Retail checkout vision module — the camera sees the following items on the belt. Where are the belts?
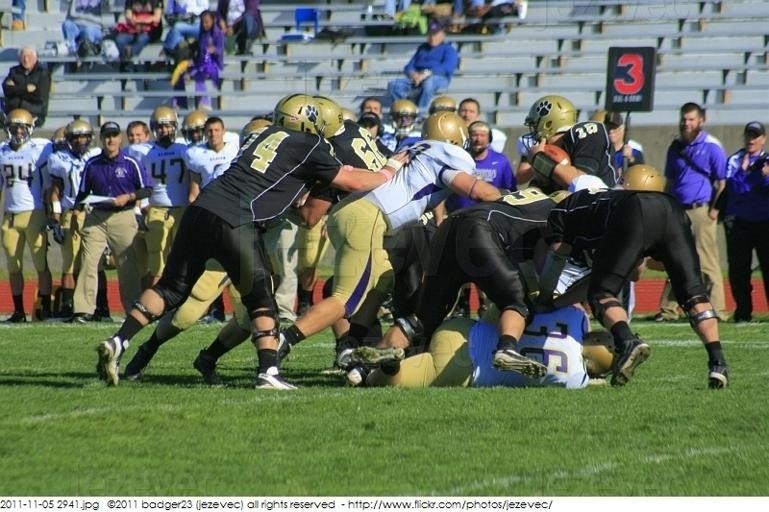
[681,202,709,209]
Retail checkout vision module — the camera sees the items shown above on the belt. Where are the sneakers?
[124,370,142,381]
[253,330,300,392]
[332,343,406,386]
[192,348,225,387]
[0,310,115,324]
[707,361,730,389]
[652,312,753,323]
[490,347,550,379]
[95,334,131,388]
[609,337,652,388]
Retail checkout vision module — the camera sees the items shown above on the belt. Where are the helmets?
[581,329,618,379]
[51,119,96,142]
[466,119,494,151]
[390,98,420,118]
[522,94,580,142]
[591,110,625,131]
[148,105,179,130]
[421,94,471,151]
[271,92,343,141]
[237,118,274,149]
[613,163,666,196]
[180,110,208,132]
[3,108,34,126]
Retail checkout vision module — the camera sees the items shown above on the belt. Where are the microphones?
[480,144,491,152]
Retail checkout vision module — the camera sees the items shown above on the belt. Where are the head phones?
[357,112,384,137]
[467,120,493,142]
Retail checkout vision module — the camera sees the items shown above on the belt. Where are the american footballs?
[544,143,572,168]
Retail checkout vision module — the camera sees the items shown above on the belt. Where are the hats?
[356,111,381,125]
[100,122,120,135]
[428,21,441,33]
[745,120,766,137]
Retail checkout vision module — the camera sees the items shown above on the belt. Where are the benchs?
[0,1,769,132]
